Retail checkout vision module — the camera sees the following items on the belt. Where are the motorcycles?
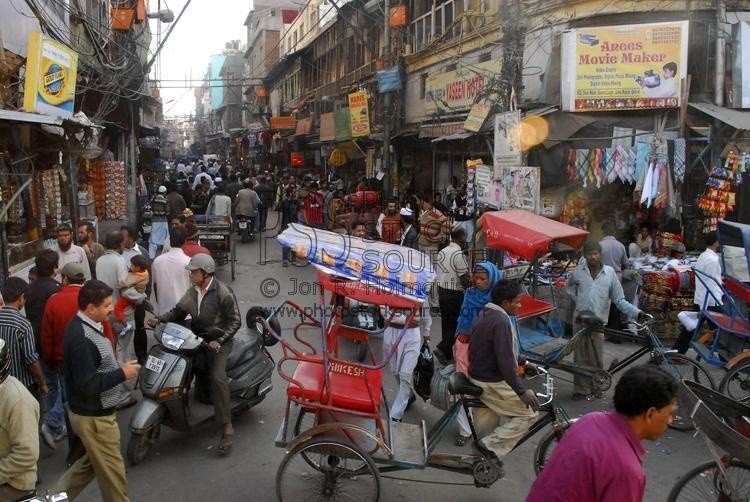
[17,479,70,502]
[234,201,263,244]
[123,298,280,468]
[140,207,152,243]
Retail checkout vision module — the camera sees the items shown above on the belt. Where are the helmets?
[184,253,215,274]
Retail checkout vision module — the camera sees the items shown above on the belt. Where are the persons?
[523,363,678,501]
[640,63,678,97]
[45,278,143,500]
[0,337,41,500]
[1,159,727,467]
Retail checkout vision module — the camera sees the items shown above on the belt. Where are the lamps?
[149,8,173,26]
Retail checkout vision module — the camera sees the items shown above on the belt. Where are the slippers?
[219,434,234,455]
[472,439,506,472]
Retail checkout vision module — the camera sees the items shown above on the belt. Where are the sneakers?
[434,349,455,366]
[39,423,68,450]
[120,322,132,336]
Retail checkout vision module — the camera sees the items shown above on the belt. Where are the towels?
[630,158,679,211]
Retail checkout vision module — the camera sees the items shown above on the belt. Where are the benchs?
[188,214,231,240]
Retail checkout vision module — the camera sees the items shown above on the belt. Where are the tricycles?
[194,214,239,282]
[264,207,750,502]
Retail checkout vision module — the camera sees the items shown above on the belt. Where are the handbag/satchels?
[431,364,456,411]
[413,341,434,402]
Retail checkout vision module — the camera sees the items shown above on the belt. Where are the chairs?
[503,272,556,327]
[689,268,750,340]
[260,298,383,415]
[714,221,750,305]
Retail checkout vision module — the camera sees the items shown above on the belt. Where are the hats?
[185,223,200,240]
[56,262,85,279]
[215,177,222,182]
[399,208,412,215]
[159,185,166,193]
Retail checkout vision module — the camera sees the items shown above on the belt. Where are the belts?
[389,322,419,329]
